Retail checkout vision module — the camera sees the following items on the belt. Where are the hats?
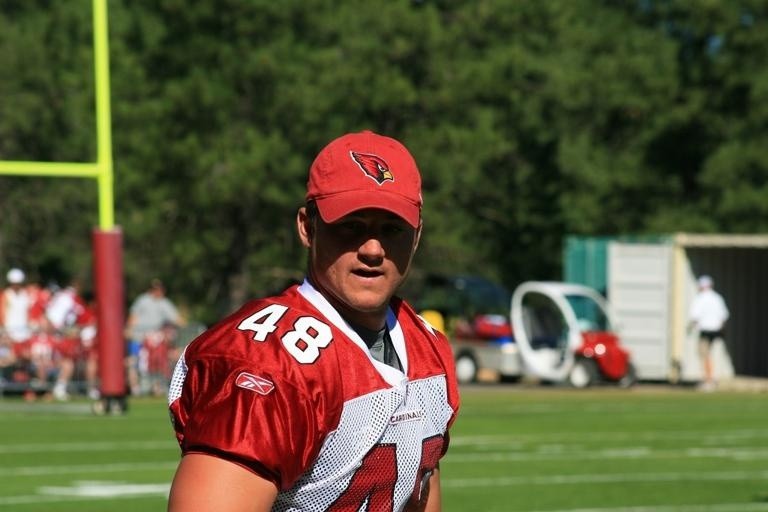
[697,274,714,289]
[6,267,27,285]
[304,128,424,229]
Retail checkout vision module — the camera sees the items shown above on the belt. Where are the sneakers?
[695,380,723,393]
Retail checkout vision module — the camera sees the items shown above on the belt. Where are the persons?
[686,276,729,389]
[0,265,182,406]
[170,130,461,512]
